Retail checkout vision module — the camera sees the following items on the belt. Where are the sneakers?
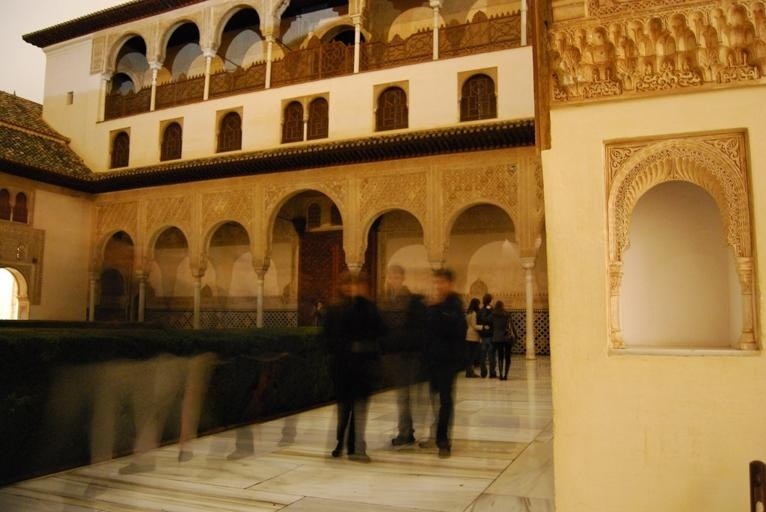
[391,432,416,446]
[418,438,451,459]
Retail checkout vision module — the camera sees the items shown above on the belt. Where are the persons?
[319,264,467,465]
[465,293,516,380]
[84,329,298,499]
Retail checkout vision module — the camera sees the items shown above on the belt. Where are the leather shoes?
[465,372,507,380]
[331,439,367,459]
